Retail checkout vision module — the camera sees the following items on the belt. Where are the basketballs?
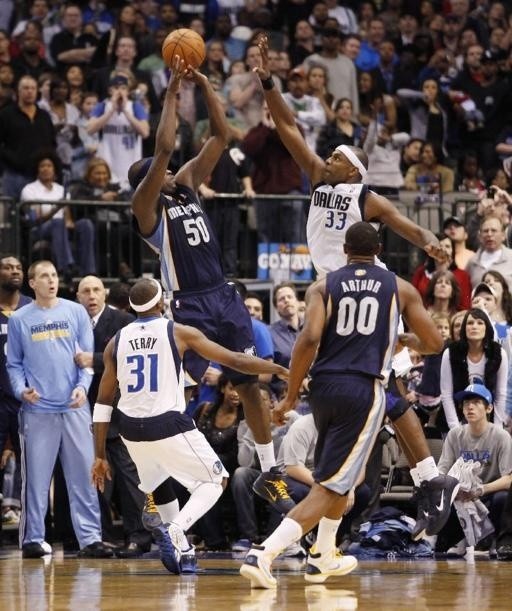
[161,28,206,73]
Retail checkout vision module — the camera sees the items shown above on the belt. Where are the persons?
[1,2,512,590]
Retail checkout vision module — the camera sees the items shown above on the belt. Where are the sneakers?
[231,538,255,552]
[419,470,462,536]
[76,541,114,560]
[141,490,164,533]
[445,538,469,558]
[252,462,297,514]
[151,520,186,575]
[180,543,206,573]
[305,530,317,545]
[305,585,358,611]
[239,541,278,591]
[114,538,151,559]
[1,510,21,530]
[21,540,46,558]
[494,543,512,561]
[411,485,431,544]
[284,541,307,558]
[304,542,359,583]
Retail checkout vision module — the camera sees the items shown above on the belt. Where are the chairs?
[379,437,400,499]
[384,439,445,501]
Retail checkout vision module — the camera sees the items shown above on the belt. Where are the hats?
[107,66,137,89]
[471,282,498,302]
[455,383,493,406]
[479,48,498,64]
[287,67,306,82]
[442,215,468,233]
